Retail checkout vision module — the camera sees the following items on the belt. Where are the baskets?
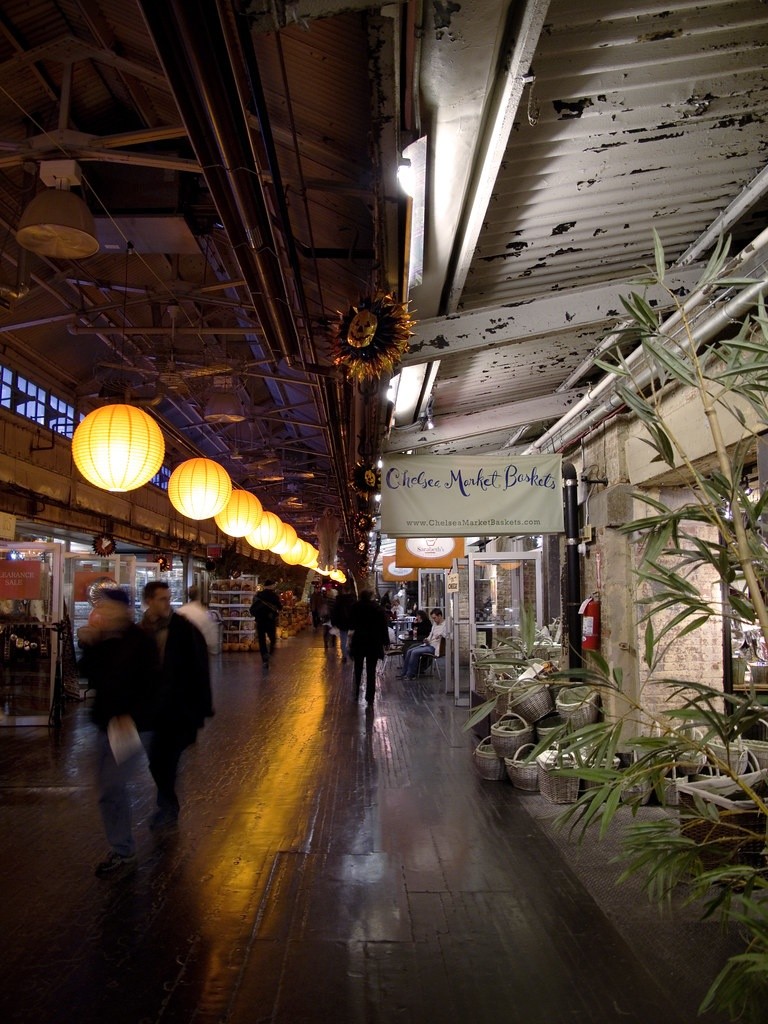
[677,768,768,894]
[472,636,722,805]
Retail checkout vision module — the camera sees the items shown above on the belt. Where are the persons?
[381,583,407,628]
[0,600,75,718]
[310,585,359,664]
[77,580,219,875]
[249,580,283,670]
[396,608,446,681]
[350,590,389,704]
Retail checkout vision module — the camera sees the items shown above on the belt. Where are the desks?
[399,634,425,641]
[392,621,417,631]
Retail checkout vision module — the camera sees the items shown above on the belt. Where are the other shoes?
[365,703,374,712]
[395,674,416,680]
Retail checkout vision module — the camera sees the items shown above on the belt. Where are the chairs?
[377,636,447,682]
[404,616,417,631]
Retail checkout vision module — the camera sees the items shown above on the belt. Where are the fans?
[98,305,233,395]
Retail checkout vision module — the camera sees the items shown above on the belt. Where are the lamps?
[419,406,434,432]
[281,539,347,583]
[268,523,297,555]
[245,511,284,550]
[167,423,232,520]
[213,490,263,537]
[257,449,284,481]
[70,239,164,492]
[16,160,100,259]
[203,373,246,422]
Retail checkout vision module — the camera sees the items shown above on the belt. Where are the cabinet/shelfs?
[208,578,255,644]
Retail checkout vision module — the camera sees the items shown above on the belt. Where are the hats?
[263,578,274,586]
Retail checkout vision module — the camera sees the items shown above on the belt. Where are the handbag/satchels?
[732,652,745,684]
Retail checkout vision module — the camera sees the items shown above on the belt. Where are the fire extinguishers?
[582,591,601,650]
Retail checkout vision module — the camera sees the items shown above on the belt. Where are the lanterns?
[245,512,347,583]
[214,490,263,538]
[71,404,166,493]
[168,458,232,521]
